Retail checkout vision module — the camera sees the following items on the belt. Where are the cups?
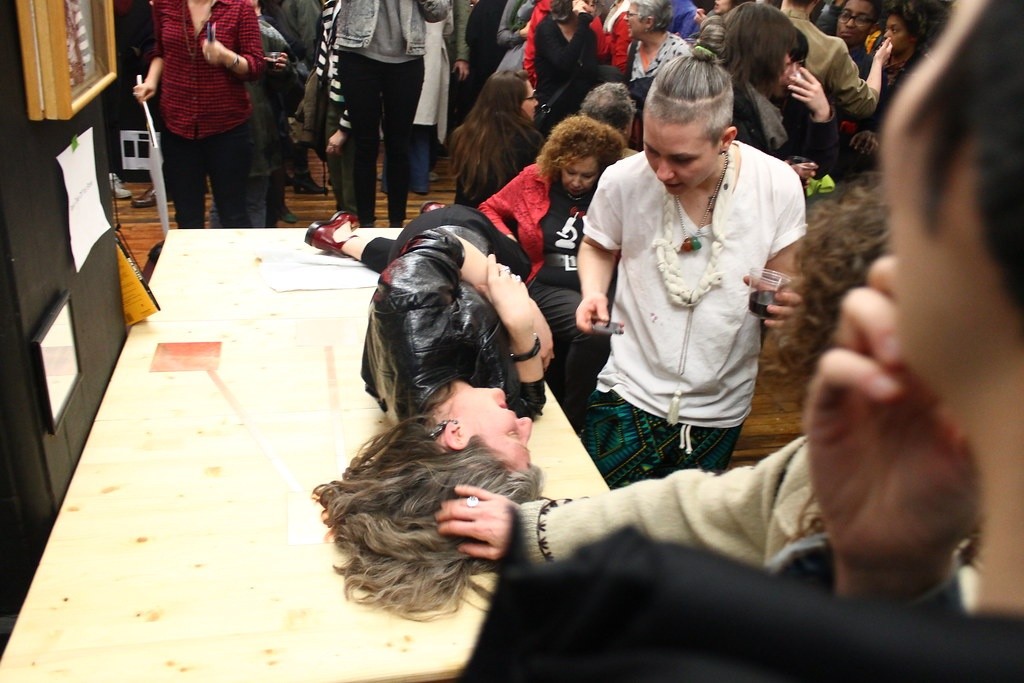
[747,267,791,321]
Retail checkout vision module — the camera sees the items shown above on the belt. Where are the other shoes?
[277,203,297,224]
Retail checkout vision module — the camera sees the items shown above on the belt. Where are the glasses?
[838,10,874,28]
[626,11,639,18]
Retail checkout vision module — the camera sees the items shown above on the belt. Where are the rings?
[467,495,479,508]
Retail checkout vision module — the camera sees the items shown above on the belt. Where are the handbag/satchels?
[624,76,655,114]
[531,88,551,134]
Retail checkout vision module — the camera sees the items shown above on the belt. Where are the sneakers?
[109,173,131,200]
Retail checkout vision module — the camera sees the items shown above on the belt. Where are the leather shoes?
[130,186,170,208]
[306,209,361,258]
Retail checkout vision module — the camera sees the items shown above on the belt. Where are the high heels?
[292,172,328,195]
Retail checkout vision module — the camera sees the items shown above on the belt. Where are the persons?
[304,202,556,621]
[432,176,986,590]
[108,0,1024,492]
[441,0,1022,683]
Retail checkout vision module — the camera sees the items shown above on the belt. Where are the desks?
[2,224,630,681]
